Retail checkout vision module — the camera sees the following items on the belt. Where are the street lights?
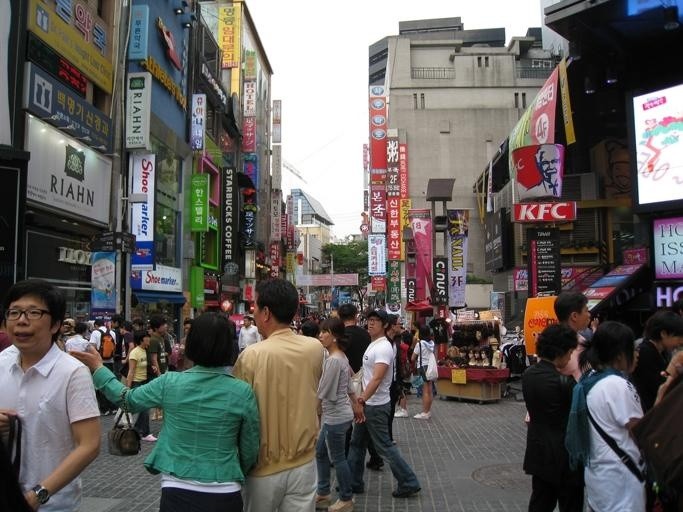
[422,176,455,258]
[402,224,417,279]
[115,187,148,315]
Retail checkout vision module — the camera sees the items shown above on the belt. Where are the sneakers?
[392,489,421,497]
[141,434,157,441]
[394,409,409,417]
[413,412,432,420]
[367,462,383,467]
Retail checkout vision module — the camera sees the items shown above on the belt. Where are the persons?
[2,280,101,511]
[335,309,421,499]
[69,312,260,510]
[459,338,502,368]
[126,330,159,442]
[55,314,194,415]
[411,325,436,419]
[232,278,323,511]
[316,319,365,511]
[522,145,562,198]
[384,313,400,446]
[237,316,262,353]
[290,312,332,337]
[521,291,683,512]
[335,304,384,470]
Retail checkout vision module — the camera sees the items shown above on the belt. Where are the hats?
[367,310,388,322]
[489,338,499,346]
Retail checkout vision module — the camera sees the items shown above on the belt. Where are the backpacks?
[95,328,115,360]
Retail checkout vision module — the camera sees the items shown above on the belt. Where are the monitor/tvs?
[624,75,683,213]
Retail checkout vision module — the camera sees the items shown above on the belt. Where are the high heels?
[315,493,331,509]
[328,499,354,512]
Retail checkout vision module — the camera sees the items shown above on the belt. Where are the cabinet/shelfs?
[434,367,510,404]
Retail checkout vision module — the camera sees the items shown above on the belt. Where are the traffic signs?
[85,231,113,252]
[120,232,136,252]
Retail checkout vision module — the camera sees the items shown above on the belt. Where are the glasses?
[3,308,52,320]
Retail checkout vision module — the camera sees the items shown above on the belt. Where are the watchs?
[32,484,50,504]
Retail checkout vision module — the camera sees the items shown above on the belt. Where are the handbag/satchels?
[419,352,439,382]
[108,388,139,456]
[0,414,34,511]
